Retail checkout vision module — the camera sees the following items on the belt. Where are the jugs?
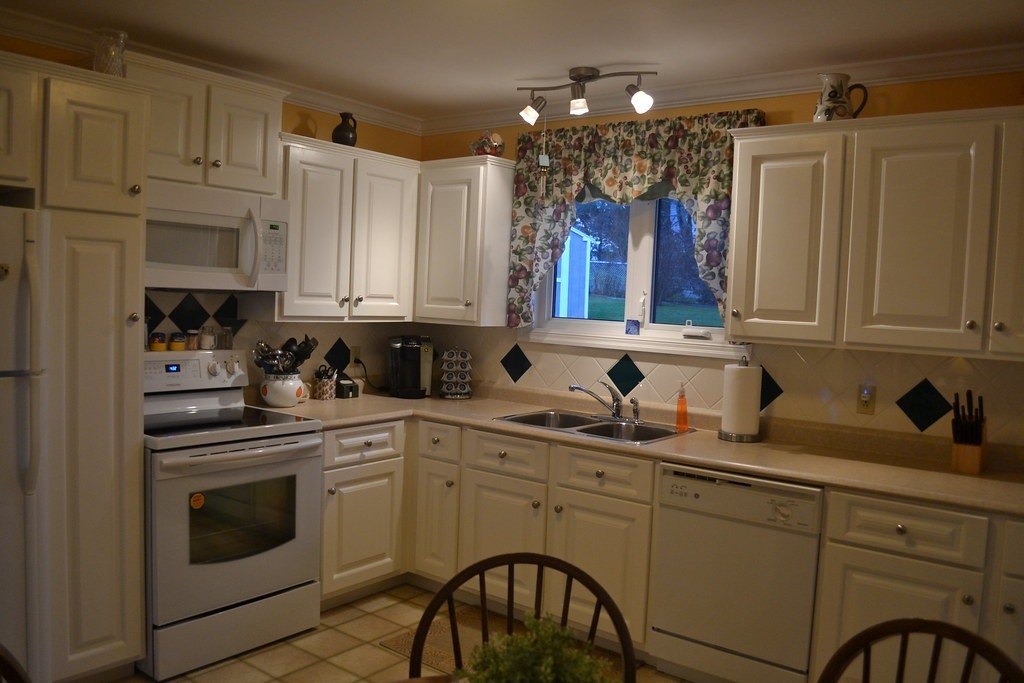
[332,113,357,147]
[94,29,127,79]
[813,73,868,123]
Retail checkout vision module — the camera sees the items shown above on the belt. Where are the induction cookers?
[142,352,323,450]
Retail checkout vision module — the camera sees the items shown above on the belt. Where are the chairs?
[815,619,1023,683]
[412,554,635,683]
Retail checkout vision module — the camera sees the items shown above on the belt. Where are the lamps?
[516,67,658,126]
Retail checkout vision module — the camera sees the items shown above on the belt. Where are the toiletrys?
[676,381,688,434]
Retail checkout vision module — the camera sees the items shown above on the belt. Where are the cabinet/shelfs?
[817,486,1023,683]
[413,155,517,328]
[409,418,462,587]
[121,49,288,198]
[850,104,1024,364]
[463,426,658,654]
[17,211,147,683]
[321,420,409,602]
[237,128,421,324]
[724,120,851,351]
[37,53,149,219]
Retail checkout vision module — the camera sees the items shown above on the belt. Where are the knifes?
[952,389,984,444]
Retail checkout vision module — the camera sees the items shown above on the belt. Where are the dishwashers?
[646,460,824,683]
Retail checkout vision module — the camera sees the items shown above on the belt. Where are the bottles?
[219,327,233,349]
[677,382,688,433]
[187,330,199,350]
[169,333,185,351]
[201,326,217,349]
[261,373,303,407]
[151,332,167,351]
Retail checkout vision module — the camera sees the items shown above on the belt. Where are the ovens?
[145,432,323,681]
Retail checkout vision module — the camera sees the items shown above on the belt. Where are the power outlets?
[856,384,876,415]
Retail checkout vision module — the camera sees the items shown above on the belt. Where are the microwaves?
[143,206,288,292]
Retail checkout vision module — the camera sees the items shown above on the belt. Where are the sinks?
[576,424,678,446]
[491,408,606,428]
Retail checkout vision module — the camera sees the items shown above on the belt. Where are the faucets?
[569,384,615,414]
[629,396,639,421]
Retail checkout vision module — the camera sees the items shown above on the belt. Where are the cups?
[313,378,336,399]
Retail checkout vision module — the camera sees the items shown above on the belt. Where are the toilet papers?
[720,364,763,435]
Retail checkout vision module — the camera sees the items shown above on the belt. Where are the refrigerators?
[0,205,82,681]
